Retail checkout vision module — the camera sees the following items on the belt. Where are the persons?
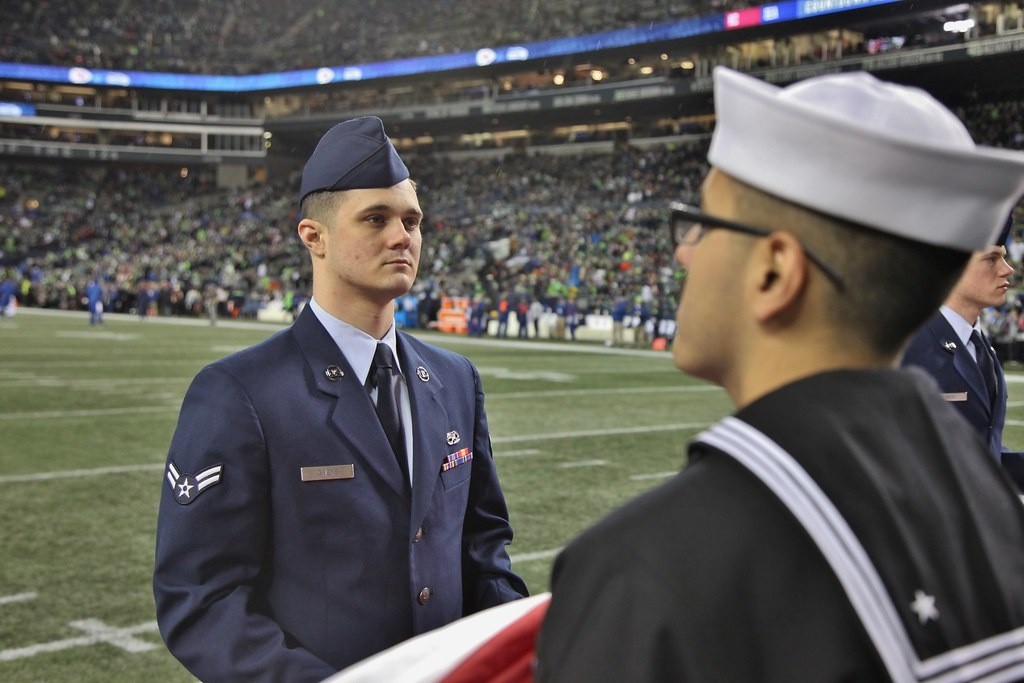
[536,62,1024,683]
[901,206,1014,470]
[153,120,532,683]
[0,0,1024,371]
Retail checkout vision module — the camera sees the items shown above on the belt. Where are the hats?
[996,206,1013,246]
[702,64,1024,254]
[281,116,411,210]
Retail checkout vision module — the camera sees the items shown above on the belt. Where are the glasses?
[667,199,845,295]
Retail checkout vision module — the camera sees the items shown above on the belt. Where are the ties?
[373,343,410,482]
[970,329,998,414]
